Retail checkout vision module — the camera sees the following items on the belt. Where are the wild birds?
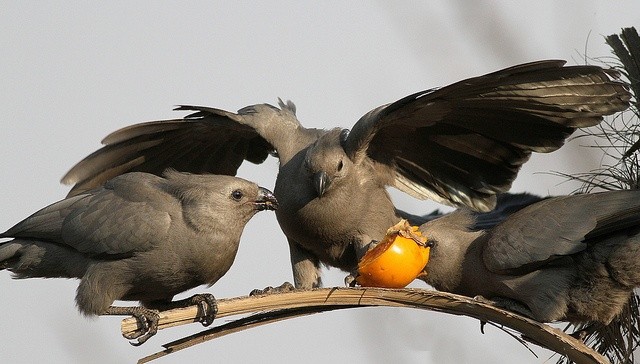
[413,186,639,337]
[57,59,634,296]
[0,168,279,349]
